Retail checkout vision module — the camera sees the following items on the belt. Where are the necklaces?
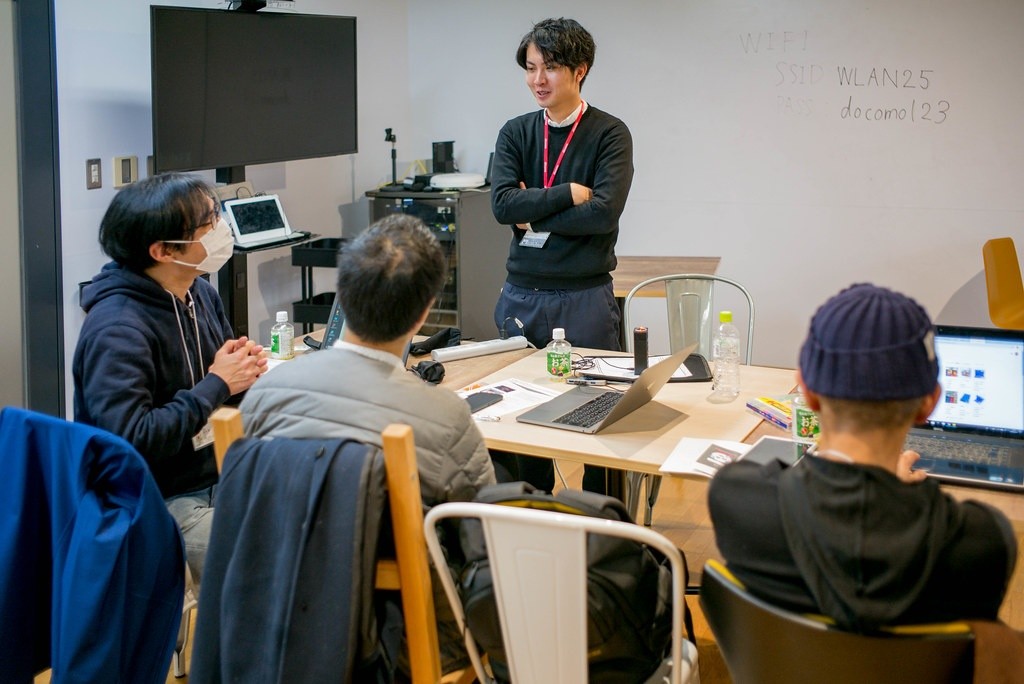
[814,449,852,463]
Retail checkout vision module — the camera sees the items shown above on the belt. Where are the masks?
[158,218,235,272]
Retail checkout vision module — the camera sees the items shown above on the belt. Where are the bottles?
[546,327,572,379]
[270,311,296,360]
[791,383,822,441]
[710,310,741,401]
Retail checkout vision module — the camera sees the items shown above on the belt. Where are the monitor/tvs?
[149,5,358,175]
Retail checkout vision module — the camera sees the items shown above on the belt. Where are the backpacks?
[458,479,688,684]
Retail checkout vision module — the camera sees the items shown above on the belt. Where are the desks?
[610,255,723,351]
[242,317,1024,633]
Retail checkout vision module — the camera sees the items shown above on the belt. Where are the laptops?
[319,289,412,368]
[901,325,1024,493]
[516,342,700,434]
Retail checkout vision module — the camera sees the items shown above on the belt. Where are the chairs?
[0,407,201,684]
[697,556,1023,684]
[983,237,1024,329]
[208,407,489,683]
[624,271,752,529]
[424,501,699,684]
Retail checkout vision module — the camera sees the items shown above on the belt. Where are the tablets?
[224,195,292,245]
[739,435,817,469]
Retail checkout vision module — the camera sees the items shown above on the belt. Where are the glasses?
[175,196,219,238]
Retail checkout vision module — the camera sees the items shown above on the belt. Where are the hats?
[799,283,940,402]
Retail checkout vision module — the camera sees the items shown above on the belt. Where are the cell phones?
[465,392,503,412]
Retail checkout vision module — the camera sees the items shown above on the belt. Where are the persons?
[72,171,268,583]
[491,16,634,349]
[240,209,496,672]
[708,281,1019,634]
